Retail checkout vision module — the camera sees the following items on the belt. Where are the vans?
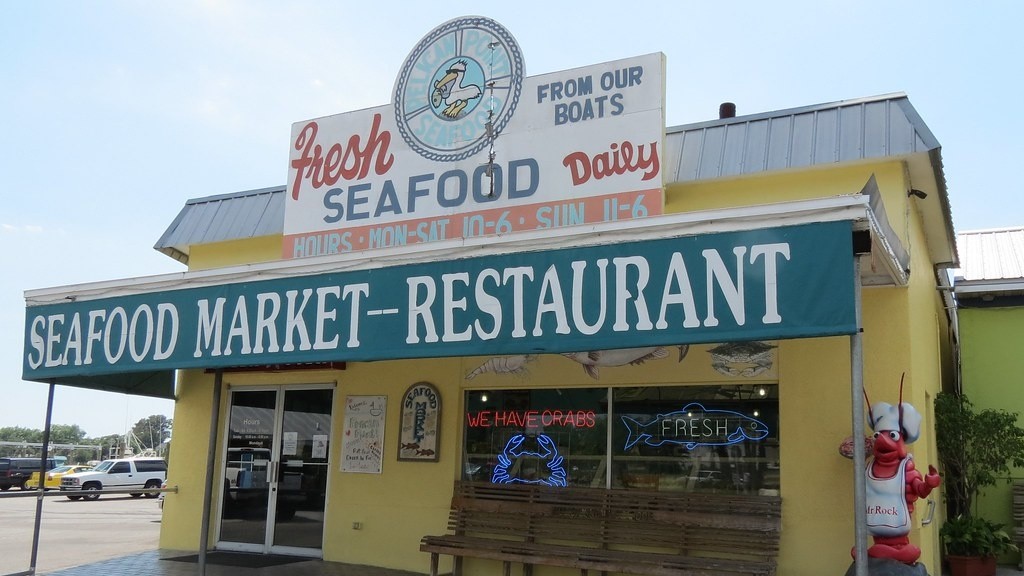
[54,456,68,467]
[0,457,56,491]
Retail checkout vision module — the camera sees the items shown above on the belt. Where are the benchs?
[1012,482,1024,571]
[419,481,781,576]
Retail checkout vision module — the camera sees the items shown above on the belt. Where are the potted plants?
[940,514,1021,575]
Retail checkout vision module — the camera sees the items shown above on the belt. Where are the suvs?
[222,446,303,514]
[59,456,168,501]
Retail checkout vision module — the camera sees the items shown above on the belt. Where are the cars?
[686,470,723,488]
[25,460,101,491]
[465,461,500,481]
